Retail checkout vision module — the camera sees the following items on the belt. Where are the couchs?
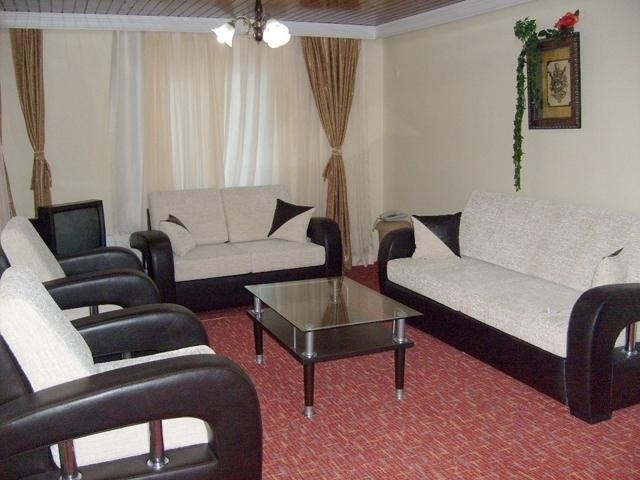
[128,182,344,315]
[375,184,640,428]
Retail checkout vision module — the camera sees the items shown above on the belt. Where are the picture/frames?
[526,31,582,132]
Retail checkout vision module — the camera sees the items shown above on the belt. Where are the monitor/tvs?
[28,199,106,258]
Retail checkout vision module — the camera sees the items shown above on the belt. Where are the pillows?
[159,211,196,257]
[0,263,96,394]
[264,195,315,245]
[407,210,464,260]
[588,244,629,290]
[1,212,67,284]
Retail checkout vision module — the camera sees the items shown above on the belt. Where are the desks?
[371,221,413,249]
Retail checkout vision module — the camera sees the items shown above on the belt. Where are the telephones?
[380,210,408,222]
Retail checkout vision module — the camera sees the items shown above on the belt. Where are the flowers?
[550,9,579,34]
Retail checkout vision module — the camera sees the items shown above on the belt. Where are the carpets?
[198,263,639,479]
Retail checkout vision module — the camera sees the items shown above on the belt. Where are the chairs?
[1,246,165,324]
[1,293,268,480]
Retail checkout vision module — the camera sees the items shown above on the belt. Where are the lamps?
[210,0,293,52]
